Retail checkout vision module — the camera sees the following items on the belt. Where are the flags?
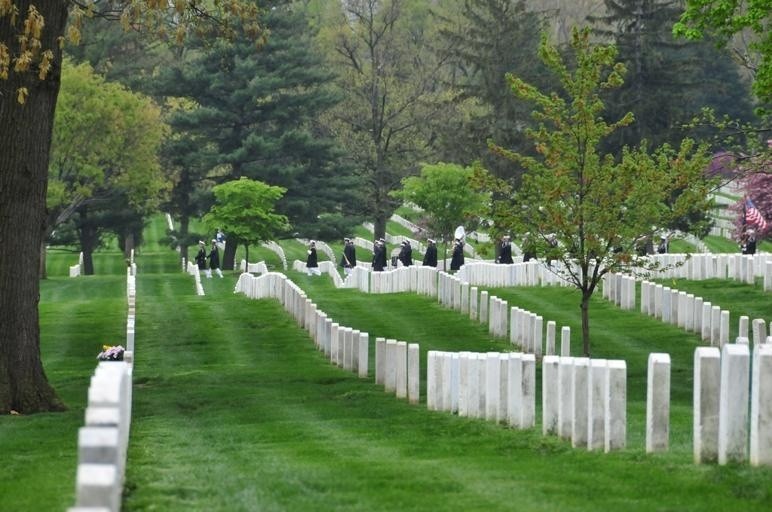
[741,194,769,236]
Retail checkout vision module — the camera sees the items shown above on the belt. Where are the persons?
[192,239,211,279]
[216,230,227,250]
[204,238,225,279]
[339,237,465,276]
[494,229,670,265]
[737,219,760,254]
[306,242,321,277]
